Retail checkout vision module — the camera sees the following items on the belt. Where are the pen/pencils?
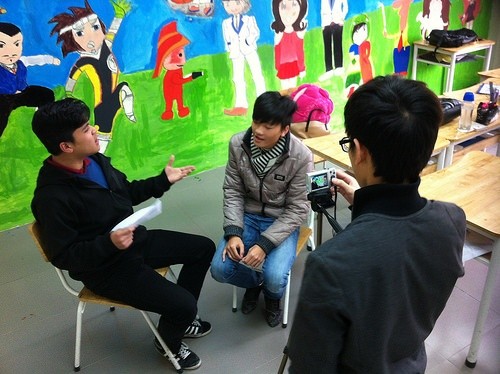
[489,81,494,103]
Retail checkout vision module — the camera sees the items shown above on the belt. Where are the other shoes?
[264,295,280,327]
[242,285,262,313]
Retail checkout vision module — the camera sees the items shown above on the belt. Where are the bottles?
[459,91,475,132]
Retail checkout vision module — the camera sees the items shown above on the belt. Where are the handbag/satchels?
[290,109,330,140]
[438,98,463,126]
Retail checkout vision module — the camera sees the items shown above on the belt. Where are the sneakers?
[184,319,212,338]
[153,339,201,370]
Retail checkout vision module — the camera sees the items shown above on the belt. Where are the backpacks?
[426,28,478,48]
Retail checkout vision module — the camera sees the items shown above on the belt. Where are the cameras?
[304,168,337,192]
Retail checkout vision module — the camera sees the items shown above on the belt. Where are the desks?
[417,150,500,368]
[438,78,500,167]
[412,39,495,93]
[477,68,500,79]
[301,132,451,251]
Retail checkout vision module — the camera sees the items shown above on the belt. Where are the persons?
[30,98,216,370]
[321,176,328,186]
[209,91,314,328]
[312,178,319,191]
[287,73,468,374]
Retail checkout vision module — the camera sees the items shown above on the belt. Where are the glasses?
[340,137,374,155]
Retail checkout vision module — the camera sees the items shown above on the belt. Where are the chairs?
[233,224,313,328]
[28,219,183,373]
